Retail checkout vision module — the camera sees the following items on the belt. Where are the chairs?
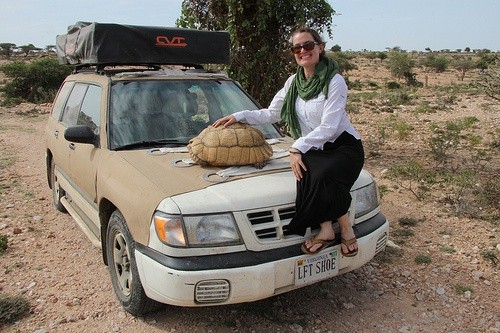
[165,92,205,138]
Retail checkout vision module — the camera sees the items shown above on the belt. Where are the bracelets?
[289,150,302,155]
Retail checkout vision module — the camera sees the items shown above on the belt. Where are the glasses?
[291,41,319,53]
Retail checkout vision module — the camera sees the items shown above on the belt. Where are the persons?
[213,29,361,255]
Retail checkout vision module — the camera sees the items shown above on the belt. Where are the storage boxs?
[55,21,230,64]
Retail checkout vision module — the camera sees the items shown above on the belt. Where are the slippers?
[339,230,359,256]
[302,233,336,254]
